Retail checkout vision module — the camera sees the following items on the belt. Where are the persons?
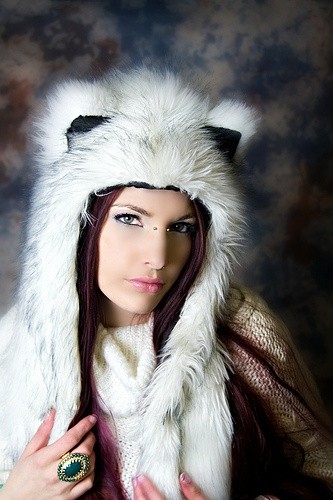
[0,68,332,499]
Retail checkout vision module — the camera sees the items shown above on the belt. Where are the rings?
[57,452,90,482]
